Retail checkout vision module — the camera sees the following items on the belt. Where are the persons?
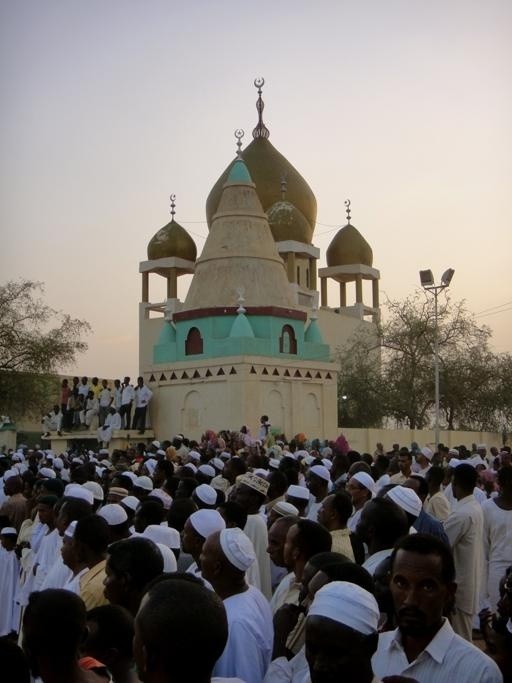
[40,377,153,449]
[0,417,512,682]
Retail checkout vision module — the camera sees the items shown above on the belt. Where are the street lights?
[418,264,456,447]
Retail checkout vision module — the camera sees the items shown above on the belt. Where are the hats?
[240,472,270,497]
[275,439,285,444]
[98,504,128,525]
[387,485,422,518]
[298,450,308,457]
[156,540,177,573]
[174,435,183,441]
[65,521,78,536]
[183,463,198,474]
[421,447,434,460]
[196,484,217,505]
[121,471,137,483]
[133,476,154,491]
[449,449,459,455]
[140,525,181,548]
[54,458,64,469]
[286,483,310,499]
[111,405,116,409]
[40,467,56,478]
[476,443,487,448]
[269,457,280,469]
[147,488,173,510]
[190,509,227,538]
[99,449,108,454]
[95,466,107,477]
[4,470,16,480]
[273,501,299,518]
[122,496,142,511]
[109,486,129,497]
[219,528,256,572]
[89,458,97,463]
[449,458,460,468]
[219,452,231,458]
[352,471,375,492]
[321,458,333,469]
[152,441,161,448]
[210,458,224,470]
[253,468,268,476]
[198,464,216,477]
[157,450,166,455]
[64,483,94,504]
[188,451,201,460]
[101,460,112,468]
[82,481,104,500]
[72,458,83,464]
[307,581,379,636]
[310,465,331,482]
[1,527,16,534]
